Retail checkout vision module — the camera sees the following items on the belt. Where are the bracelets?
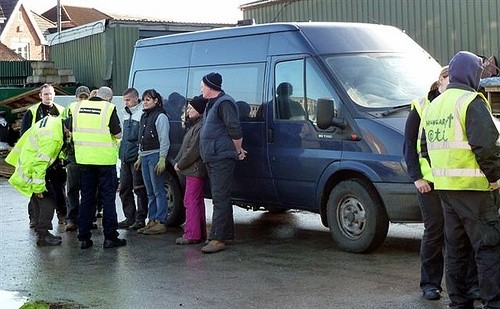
[238,152,242,156]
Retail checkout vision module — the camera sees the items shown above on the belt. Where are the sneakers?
[104,237,126,248]
[80,240,93,249]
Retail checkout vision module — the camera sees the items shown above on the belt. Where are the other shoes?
[425,289,440,300]
[144,222,167,235]
[66,222,77,231]
[128,217,145,231]
[58,220,66,233]
[205,240,233,247]
[176,234,200,244]
[118,215,134,228]
[36,231,62,246]
[202,239,226,253]
[138,222,159,234]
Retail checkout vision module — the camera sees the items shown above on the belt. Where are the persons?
[69,86,127,249]
[199,72,248,254]
[420,51,500,309]
[134,88,172,235]
[60,86,98,231]
[4,116,73,247]
[119,88,147,231]
[21,83,69,228]
[403,66,450,301]
[89,89,104,222]
[174,96,207,244]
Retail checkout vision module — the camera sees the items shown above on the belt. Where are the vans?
[127,19,500,253]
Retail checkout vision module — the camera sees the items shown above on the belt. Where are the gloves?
[154,156,166,176]
[134,155,141,171]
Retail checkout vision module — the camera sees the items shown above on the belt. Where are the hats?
[203,72,222,90]
[190,95,208,114]
[75,86,90,97]
